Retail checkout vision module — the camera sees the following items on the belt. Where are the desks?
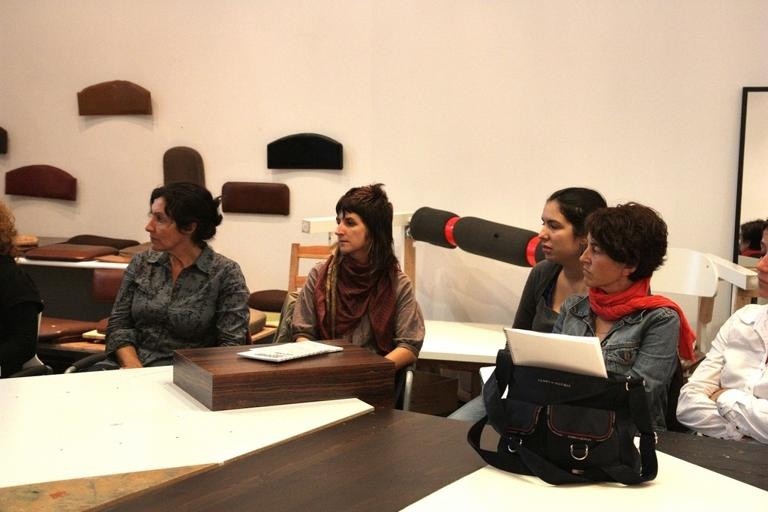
[14,256,131,322]
[1,366,768,512]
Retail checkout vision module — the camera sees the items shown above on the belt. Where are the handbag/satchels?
[468,350,658,483]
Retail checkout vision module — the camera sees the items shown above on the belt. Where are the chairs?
[650,249,718,373]
[65,244,337,374]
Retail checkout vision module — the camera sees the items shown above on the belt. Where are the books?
[236,340,344,364]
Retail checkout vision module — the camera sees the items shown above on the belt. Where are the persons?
[88,180,250,371]
[550,201,689,434]
[290,184,426,409]
[738,219,766,268]
[448,188,607,424]
[0,202,45,380]
[676,218,768,445]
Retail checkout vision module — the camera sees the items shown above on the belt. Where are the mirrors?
[733,87,768,268]
